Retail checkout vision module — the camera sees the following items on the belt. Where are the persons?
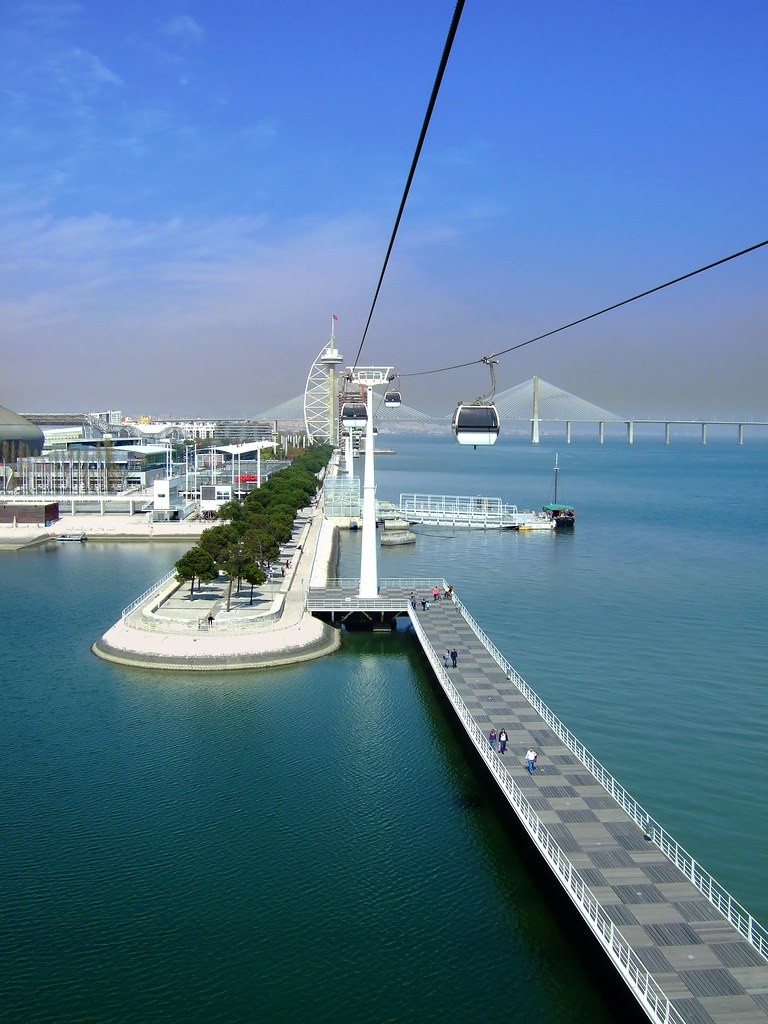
[489,728,508,754]
[280,560,291,576]
[208,613,215,628]
[443,648,457,669]
[410,585,453,611]
[525,749,537,774]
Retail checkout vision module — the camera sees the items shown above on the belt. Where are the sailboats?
[541,452,575,527]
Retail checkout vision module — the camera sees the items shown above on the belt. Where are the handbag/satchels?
[497,742,501,752]
[443,655,446,659]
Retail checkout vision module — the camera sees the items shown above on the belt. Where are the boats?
[383,519,411,531]
[380,528,417,546]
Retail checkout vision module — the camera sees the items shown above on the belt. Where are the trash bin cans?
[646,823,653,835]
[506,669,512,677]
